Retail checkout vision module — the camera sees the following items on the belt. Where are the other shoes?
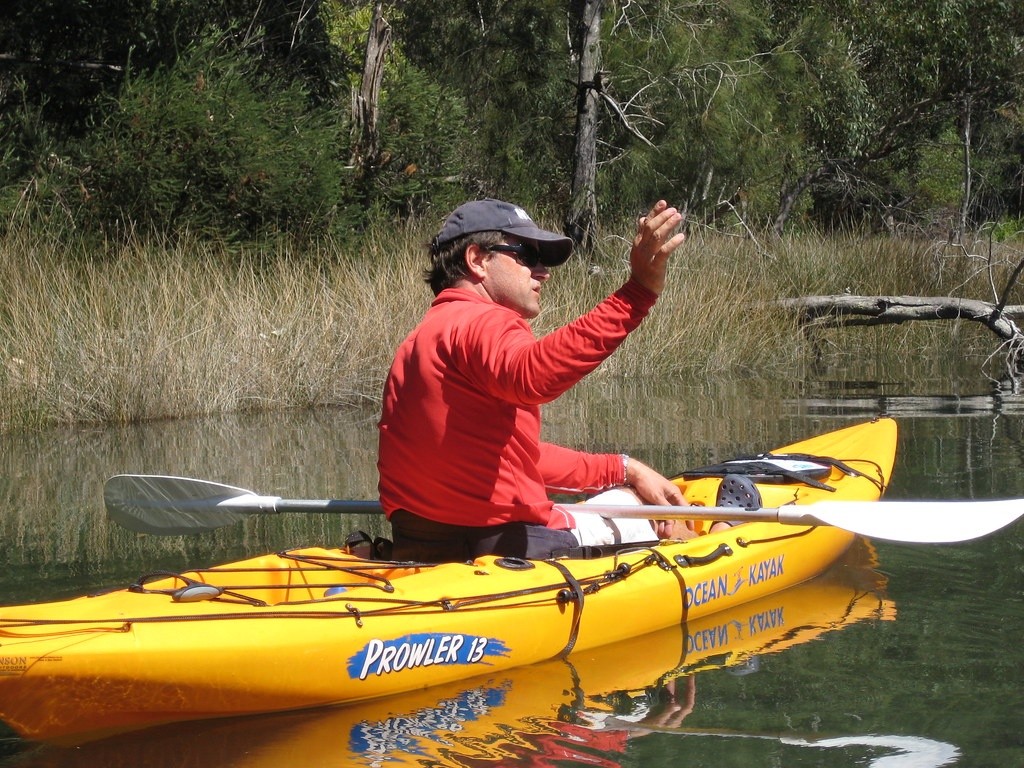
[709,474,765,533]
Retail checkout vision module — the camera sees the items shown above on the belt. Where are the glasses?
[490,242,551,268]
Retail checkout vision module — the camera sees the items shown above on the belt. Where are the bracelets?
[622,454,630,487]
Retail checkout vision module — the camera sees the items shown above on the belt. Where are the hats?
[436,197,573,267]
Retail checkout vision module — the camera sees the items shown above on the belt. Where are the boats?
[8,580,893,768]
[0,416,898,743]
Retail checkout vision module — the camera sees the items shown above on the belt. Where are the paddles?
[103,472,1024,544]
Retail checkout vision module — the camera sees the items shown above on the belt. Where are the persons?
[377,198,762,564]
